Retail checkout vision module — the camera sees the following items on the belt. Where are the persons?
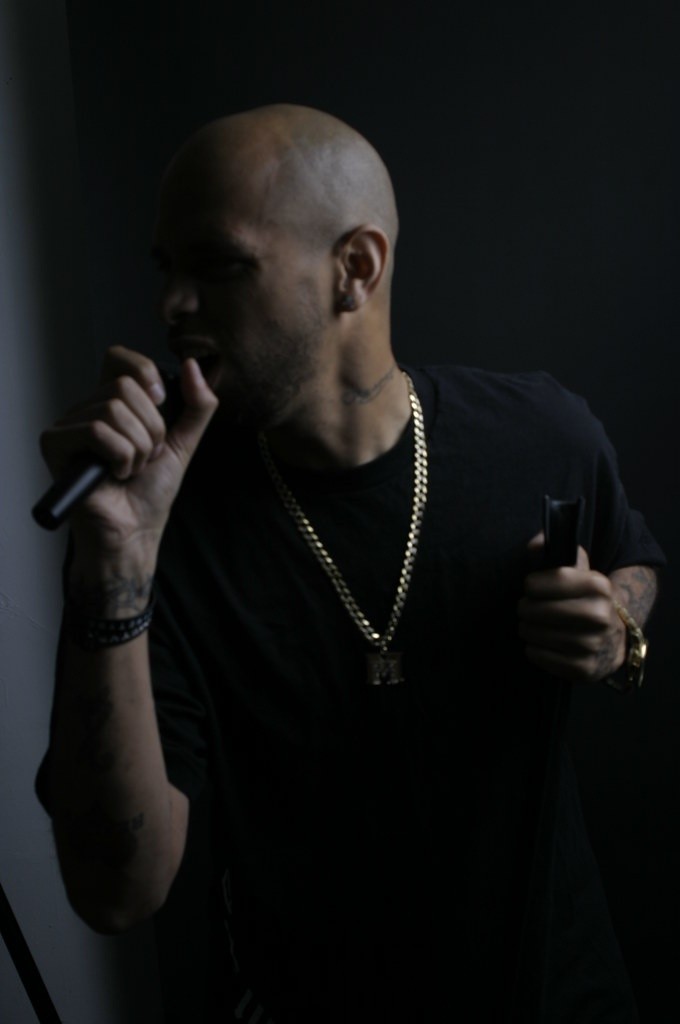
[33,104,667,1024]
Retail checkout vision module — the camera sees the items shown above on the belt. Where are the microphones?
[28,365,192,533]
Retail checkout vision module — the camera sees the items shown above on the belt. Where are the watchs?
[605,598,648,696]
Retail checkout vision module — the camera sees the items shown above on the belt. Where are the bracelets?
[61,589,157,645]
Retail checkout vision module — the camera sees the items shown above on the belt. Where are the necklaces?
[257,372,428,685]
